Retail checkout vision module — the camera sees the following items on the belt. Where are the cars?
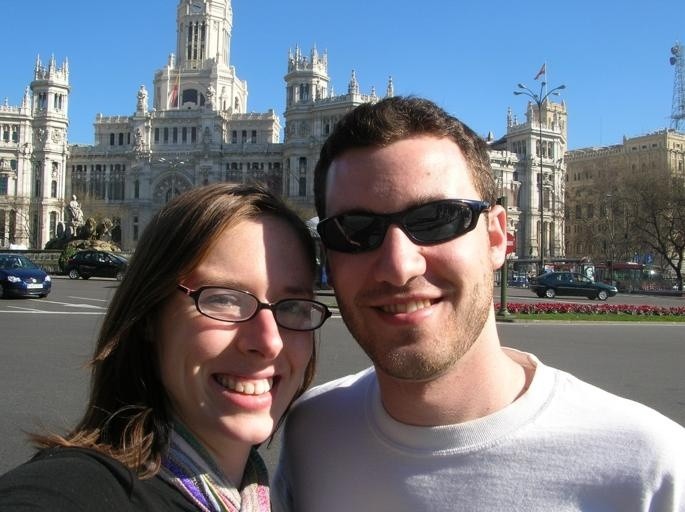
[59,249,129,281]
[528,271,619,301]
[0,253,52,299]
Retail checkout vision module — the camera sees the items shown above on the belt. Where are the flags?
[168,72,180,106]
[533,63,545,80]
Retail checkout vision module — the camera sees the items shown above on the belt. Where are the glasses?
[177,283,333,334]
[316,198,493,255]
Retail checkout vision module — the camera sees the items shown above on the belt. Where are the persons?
[68,193,83,222]
[271,95,684,512]
[0,182,332,512]
